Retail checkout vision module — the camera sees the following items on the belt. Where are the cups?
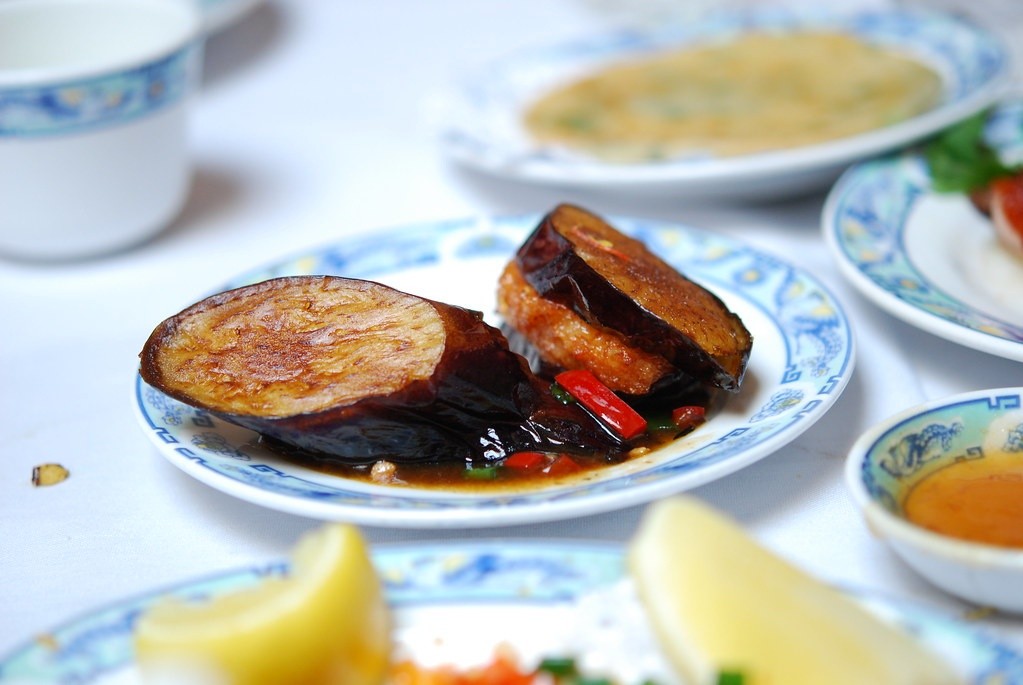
[0,1,203,261]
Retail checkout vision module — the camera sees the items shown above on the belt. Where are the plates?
[119,209,857,531]
[820,97,1022,364]
[424,0,1022,202]
[0,538,1023,684]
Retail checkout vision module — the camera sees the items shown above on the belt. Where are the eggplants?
[497,203,753,401]
[141,276,546,466]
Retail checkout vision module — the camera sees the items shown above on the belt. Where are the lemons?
[125,518,395,685]
[628,494,965,685]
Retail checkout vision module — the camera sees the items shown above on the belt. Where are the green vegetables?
[923,106,1022,193]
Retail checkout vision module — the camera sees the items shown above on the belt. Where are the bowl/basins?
[842,386,1023,617]
